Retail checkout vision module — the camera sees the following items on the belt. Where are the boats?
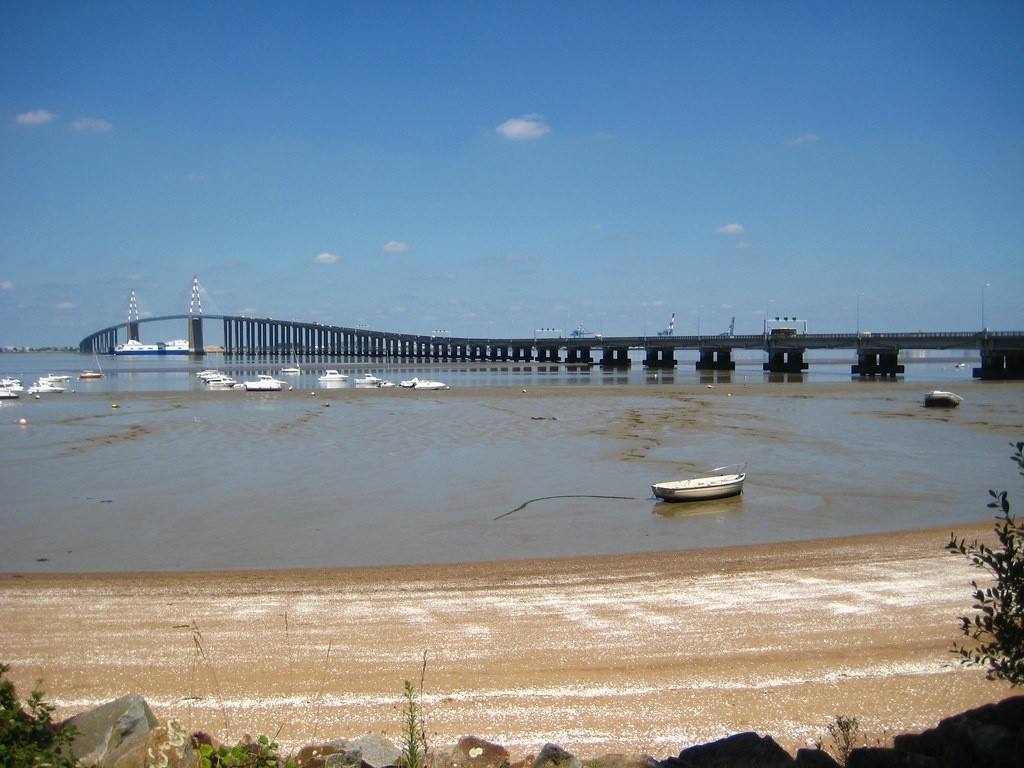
[196,369,236,386]
[923,391,964,408]
[80,369,102,379]
[112,339,189,355]
[414,380,446,389]
[1,374,72,399]
[354,374,382,383]
[401,377,421,390]
[378,381,396,388]
[317,369,350,381]
[245,375,288,390]
[651,461,748,500]
[282,367,300,372]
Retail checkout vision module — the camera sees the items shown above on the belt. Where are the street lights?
[856,293,865,333]
[766,299,774,334]
[982,284,991,331]
